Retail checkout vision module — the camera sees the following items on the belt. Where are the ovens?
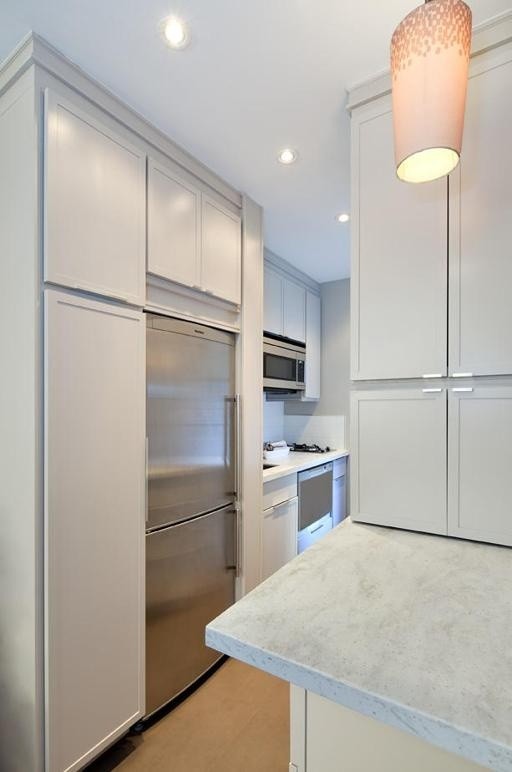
[263,336,305,392]
[297,462,333,543]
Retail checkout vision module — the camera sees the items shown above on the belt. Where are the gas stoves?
[287,443,335,454]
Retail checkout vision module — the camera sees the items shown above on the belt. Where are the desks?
[206,516,512,772]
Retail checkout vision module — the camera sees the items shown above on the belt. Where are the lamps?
[389,1,472,185]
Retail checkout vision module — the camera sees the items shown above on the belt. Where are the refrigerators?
[146,310,234,721]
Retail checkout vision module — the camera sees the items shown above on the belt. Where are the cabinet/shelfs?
[261,473,299,581]
[332,456,347,529]
[43,83,246,309]
[42,288,148,772]
[264,248,323,401]
[344,9,512,547]
